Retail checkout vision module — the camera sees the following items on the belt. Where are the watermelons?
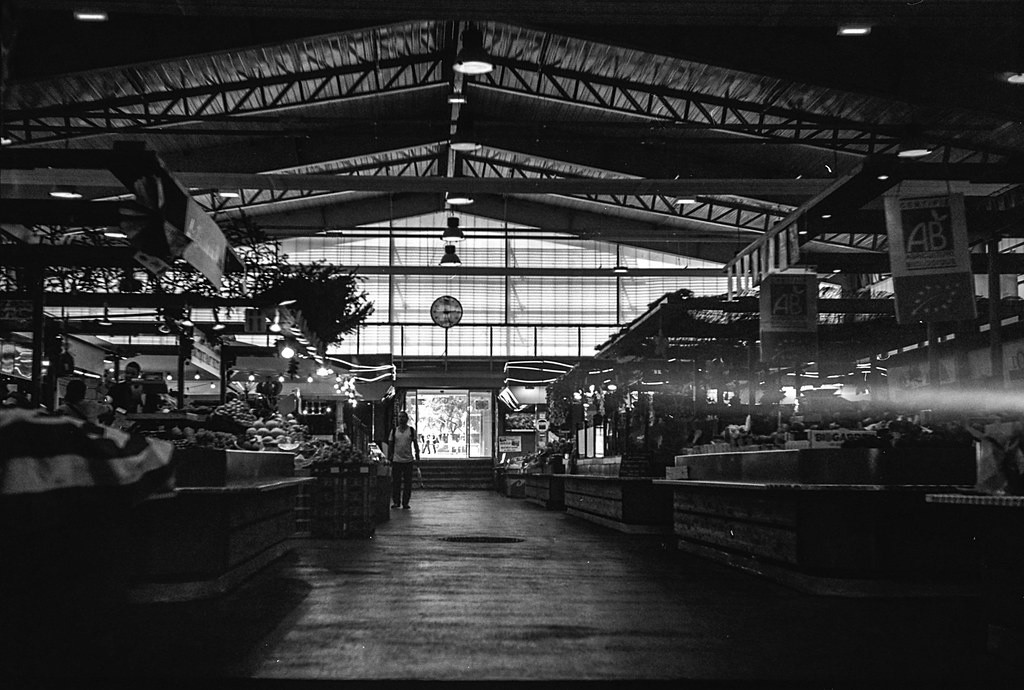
[246,420,286,437]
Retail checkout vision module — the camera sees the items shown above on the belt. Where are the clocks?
[430,296,463,328]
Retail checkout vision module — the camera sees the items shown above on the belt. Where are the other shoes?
[403,505,410,509]
[391,502,400,508]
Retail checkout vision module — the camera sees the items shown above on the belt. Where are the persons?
[387,412,420,508]
[54,380,88,421]
[102,361,143,414]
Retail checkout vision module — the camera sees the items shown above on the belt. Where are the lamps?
[182,309,194,327]
[99,306,112,325]
[446,175,474,205]
[450,112,483,151]
[439,245,462,267]
[451,27,496,74]
[212,308,225,330]
[48,184,86,200]
[440,217,466,242]
